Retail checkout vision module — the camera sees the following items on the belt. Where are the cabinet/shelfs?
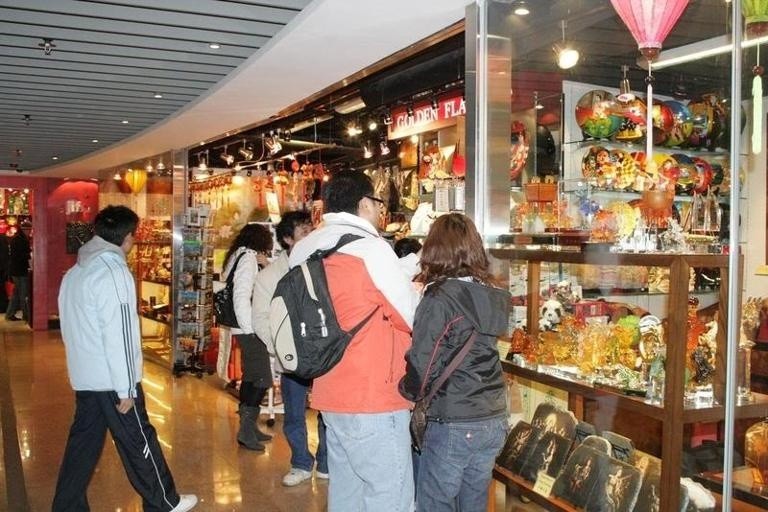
[386,115,465,239]
[132,240,174,371]
[560,78,767,331]
[175,226,215,378]
[488,245,767,512]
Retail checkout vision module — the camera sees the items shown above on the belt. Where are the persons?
[51,206,199,512]
[394,240,421,256]
[214,224,272,450]
[6,221,37,322]
[251,210,329,485]
[286,170,423,512]
[399,214,507,512]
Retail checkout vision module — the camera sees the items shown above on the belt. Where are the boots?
[237,403,265,450]
[256,429,271,440]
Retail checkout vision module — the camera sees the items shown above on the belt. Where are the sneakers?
[283,468,312,486]
[170,494,197,512]
[316,471,329,479]
[4,315,21,321]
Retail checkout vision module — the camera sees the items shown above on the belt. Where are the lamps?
[112,173,122,181]
[383,113,394,125]
[552,41,580,70]
[220,153,235,166]
[264,138,282,155]
[157,162,166,169]
[429,96,440,110]
[197,156,207,170]
[406,106,415,117]
[379,141,391,156]
[144,165,153,171]
[508,1,534,18]
[237,147,254,162]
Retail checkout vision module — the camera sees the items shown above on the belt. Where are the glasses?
[365,194,384,209]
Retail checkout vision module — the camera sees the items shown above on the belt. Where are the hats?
[21,222,32,230]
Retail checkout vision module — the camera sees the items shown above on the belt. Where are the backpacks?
[268,234,380,382]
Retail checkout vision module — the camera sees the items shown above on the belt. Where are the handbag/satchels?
[213,252,261,329]
[409,329,477,456]
